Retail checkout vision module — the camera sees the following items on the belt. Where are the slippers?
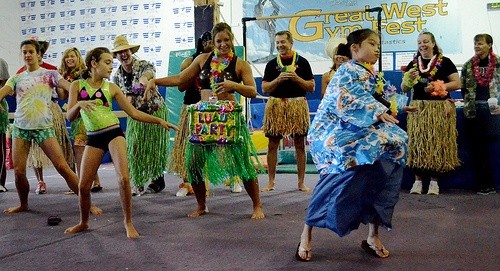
[295,242,312,262]
[91,183,103,193]
[361,240,389,258]
[261,188,275,192]
[298,187,309,192]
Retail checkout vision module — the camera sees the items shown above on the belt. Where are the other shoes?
[146,175,165,193]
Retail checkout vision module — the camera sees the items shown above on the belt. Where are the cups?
[487,98,498,115]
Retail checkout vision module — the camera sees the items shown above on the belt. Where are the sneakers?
[427,181,440,195]
[476,185,496,195]
[226,177,242,192]
[410,180,423,194]
[176,183,194,196]
[35,180,47,194]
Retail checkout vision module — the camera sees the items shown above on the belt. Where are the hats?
[20,34,49,52]
[112,36,140,59]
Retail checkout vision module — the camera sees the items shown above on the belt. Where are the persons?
[56,48,103,194]
[110,35,169,194]
[0,59,12,192]
[461,34,500,195]
[400,32,461,195]
[15,35,76,194]
[169,31,242,198]
[261,30,315,192]
[322,37,350,96]
[143,23,266,220]
[0,40,104,217]
[253,0,280,56]
[296,28,418,262]
[64,46,180,239]
